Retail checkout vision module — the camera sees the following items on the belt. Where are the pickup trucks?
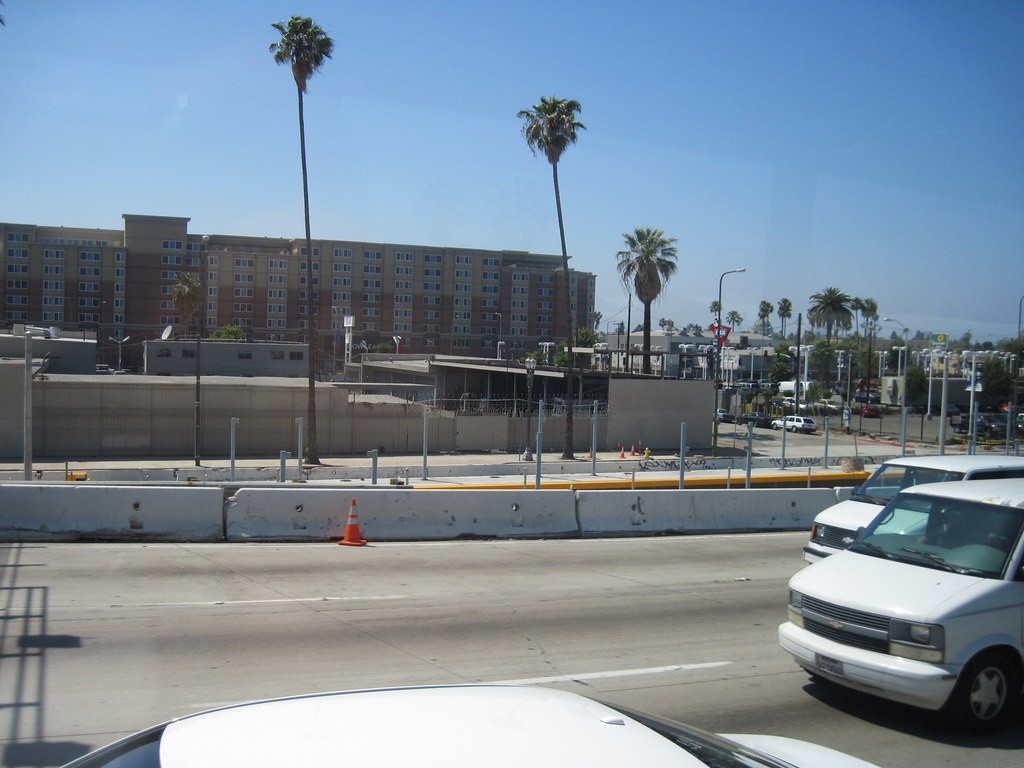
[950,413,1012,440]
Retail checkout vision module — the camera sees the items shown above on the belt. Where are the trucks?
[777,382,803,395]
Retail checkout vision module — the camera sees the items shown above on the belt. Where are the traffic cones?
[619,447,626,459]
[588,448,592,459]
[629,444,636,456]
[616,441,621,451]
[339,498,367,546]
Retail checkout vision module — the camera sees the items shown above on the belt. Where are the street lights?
[800,345,812,399]
[835,349,847,380]
[714,269,746,440]
[747,347,760,381]
[912,351,923,368]
[985,349,1017,374]
[962,350,985,455]
[892,345,906,376]
[884,317,908,433]
[875,350,887,378]
[678,344,695,379]
[699,345,714,380]
[495,312,502,342]
[721,347,735,383]
[922,348,942,420]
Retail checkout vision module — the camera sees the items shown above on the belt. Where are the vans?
[760,382,779,396]
[738,383,760,396]
[777,477,1024,737]
[801,455,1024,566]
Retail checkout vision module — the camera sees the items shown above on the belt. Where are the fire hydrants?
[644,448,651,457]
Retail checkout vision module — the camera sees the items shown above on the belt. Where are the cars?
[712,408,735,424]
[851,392,881,405]
[861,405,880,418]
[772,416,817,434]
[735,412,776,428]
[782,397,795,405]
[59,684,880,767]
